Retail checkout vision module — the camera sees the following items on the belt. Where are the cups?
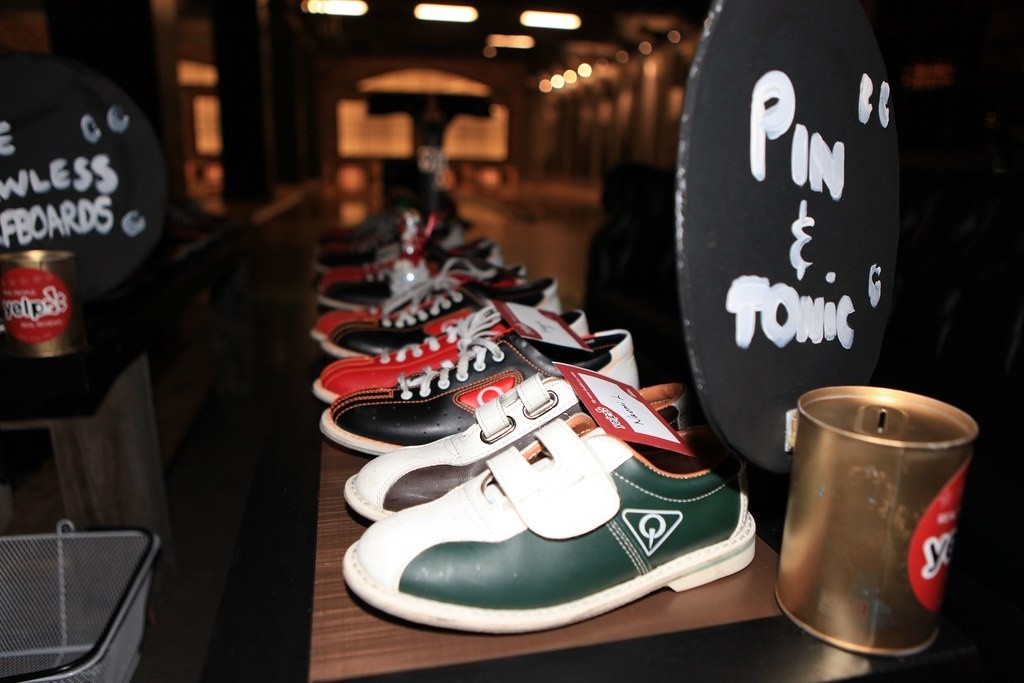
[775,383,981,661]
[1,246,91,359]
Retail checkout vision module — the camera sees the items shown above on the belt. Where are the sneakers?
[319,324,638,457]
[345,370,689,522]
[312,307,592,406]
[312,208,563,358]
[343,412,758,633]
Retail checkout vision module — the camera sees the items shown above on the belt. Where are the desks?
[307,435,979,682]
[0,228,262,554]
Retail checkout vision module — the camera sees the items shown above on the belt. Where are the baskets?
[0,520,162,683]
